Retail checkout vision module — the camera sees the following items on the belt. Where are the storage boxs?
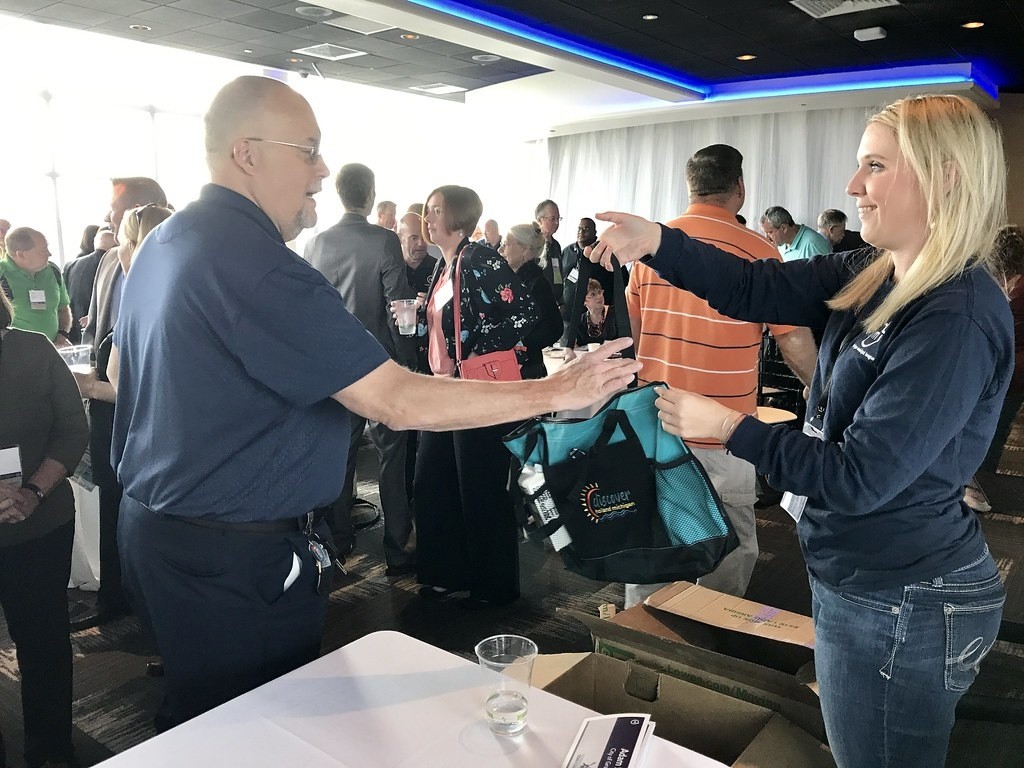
[497,649,838,768]
[554,580,830,748]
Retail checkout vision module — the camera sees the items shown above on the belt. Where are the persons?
[105,76,644,733]
[582,94,1024,768]
[0,177,178,674]
[303,163,630,609]
[0,288,90,768]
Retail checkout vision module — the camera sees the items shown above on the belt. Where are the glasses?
[231,136,321,164]
[541,216,563,222]
[577,225,593,232]
[134,202,160,238]
[586,289,605,298]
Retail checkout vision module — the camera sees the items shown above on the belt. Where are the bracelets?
[21,482,47,504]
[57,329,71,338]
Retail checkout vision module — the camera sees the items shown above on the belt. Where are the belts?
[175,507,319,534]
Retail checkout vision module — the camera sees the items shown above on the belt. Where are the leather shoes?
[145,655,165,676]
[69,602,126,629]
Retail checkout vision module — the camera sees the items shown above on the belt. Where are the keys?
[318,538,348,576]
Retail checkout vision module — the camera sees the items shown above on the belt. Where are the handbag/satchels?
[453,243,522,382]
[503,372,741,584]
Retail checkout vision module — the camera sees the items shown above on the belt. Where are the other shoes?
[385,560,417,579]
[962,485,993,514]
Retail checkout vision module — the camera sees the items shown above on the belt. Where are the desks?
[762,386,789,398]
[757,406,798,425]
[89,630,733,768]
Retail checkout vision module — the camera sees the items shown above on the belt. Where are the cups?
[474,635,539,736]
[587,343,601,352]
[57,345,94,375]
[393,299,418,335]
